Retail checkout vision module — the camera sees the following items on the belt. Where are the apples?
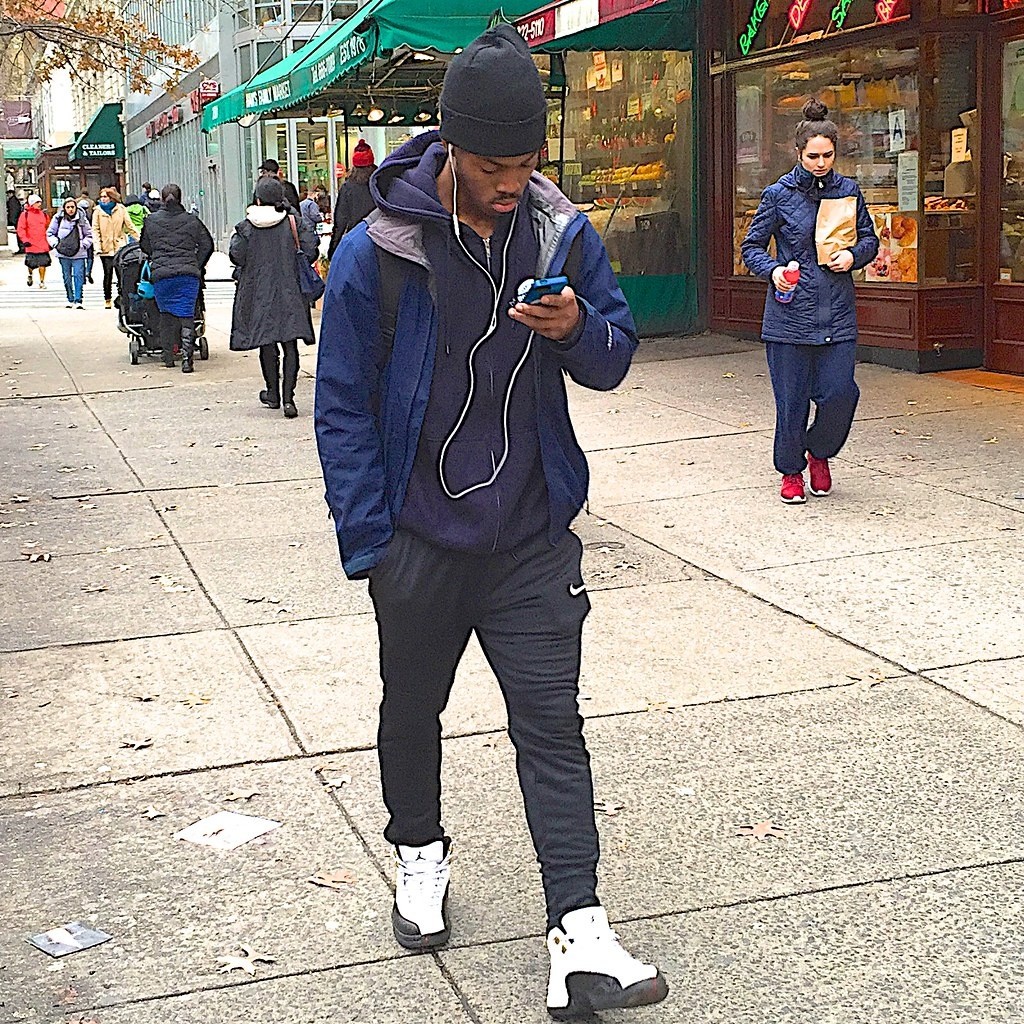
[579,160,664,185]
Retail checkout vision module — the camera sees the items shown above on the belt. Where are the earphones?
[447,143,454,163]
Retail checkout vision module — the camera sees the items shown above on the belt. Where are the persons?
[5,183,330,373]
[327,139,378,259]
[312,25,668,1017]
[229,176,319,418]
[739,96,878,503]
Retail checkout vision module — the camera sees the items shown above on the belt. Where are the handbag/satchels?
[56,222,80,257]
[288,213,326,303]
[137,259,155,299]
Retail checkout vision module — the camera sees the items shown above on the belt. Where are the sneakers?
[389,836,458,949]
[806,451,833,497]
[545,904,669,1019]
[780,472,809,504]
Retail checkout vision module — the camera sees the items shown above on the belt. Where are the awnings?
[202,0,695,131]
[68,101,126,162]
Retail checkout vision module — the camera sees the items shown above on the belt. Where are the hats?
[28,194,42,206]
[100,186,122,203]
[63,187,89,208]
[258,159,279,172]
[439,22,547,157]
[353,139,374,167]
[148,189,160,199]
[126,194,140,204]
[6,190,15,194]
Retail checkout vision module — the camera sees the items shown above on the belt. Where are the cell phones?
[523,276,568,306]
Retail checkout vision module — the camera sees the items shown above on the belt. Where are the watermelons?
[593,196,658,209]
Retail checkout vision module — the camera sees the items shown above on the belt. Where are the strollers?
[115,240,210,366]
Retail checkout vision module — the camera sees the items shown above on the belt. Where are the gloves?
[24,242,31,247]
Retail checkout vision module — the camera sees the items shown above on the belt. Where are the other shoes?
[105,300,111,309]
[182,361,193,373]
[27,273,32,286]
[39,281,46,289]
[15,249,24,254]
[166,360,175,367]
[66,301,83,309]
[283,396,297,417]
[259,390,281,409]
[88,276,93,284]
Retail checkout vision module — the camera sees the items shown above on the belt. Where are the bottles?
[775,260,799,304]
[128,292,140,300]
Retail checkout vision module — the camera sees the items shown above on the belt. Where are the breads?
[890,214,918,283]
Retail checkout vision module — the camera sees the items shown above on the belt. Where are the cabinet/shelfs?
[577,142,668,202]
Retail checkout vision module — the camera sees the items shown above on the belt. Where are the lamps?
[305,91,442,122]
